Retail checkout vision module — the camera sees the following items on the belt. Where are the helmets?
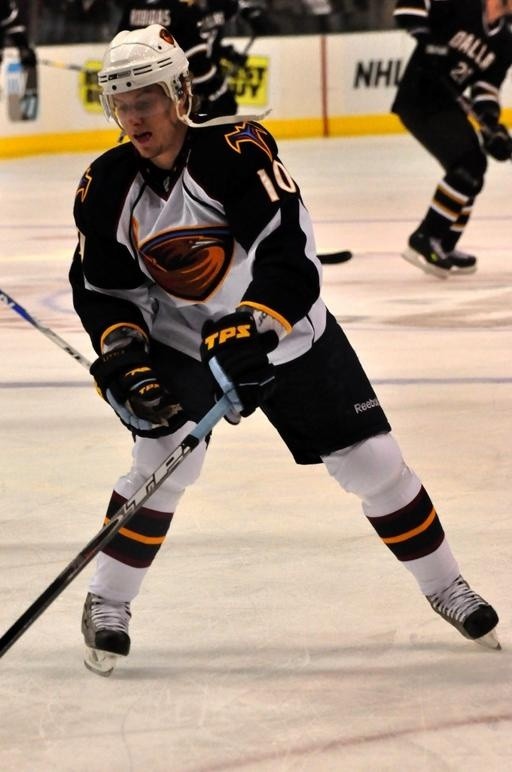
[97,23,193,120]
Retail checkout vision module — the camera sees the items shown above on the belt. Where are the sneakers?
[425,574,499,640]
[81,592,132,655]
[446,248,476,266]
[409,227,449,269]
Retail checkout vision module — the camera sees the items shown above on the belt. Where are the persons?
[67,24,502,655]
[391,0,512,270]
[1,1,39,119]
[117,0,250,122]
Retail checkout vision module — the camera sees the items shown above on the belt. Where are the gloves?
[90,340,187,438]
[223,46,247,66]
[199,312,278,425]
[480,122,512,160]
